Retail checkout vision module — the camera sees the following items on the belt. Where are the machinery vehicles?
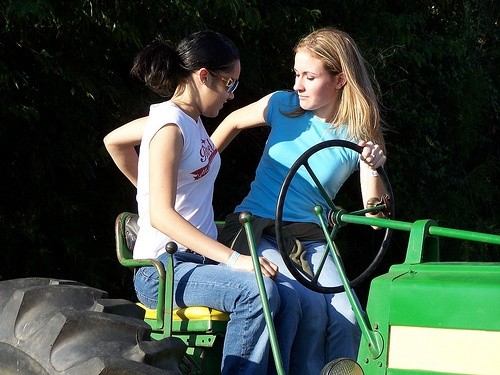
[0,139,500,375]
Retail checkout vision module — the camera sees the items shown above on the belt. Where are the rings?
[371,154,375,158]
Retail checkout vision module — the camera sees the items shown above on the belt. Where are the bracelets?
[367,164,385,176]
[225,250,241,268]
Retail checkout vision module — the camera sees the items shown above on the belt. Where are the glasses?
[196,67,239,94]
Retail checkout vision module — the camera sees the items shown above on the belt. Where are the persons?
[101,29,302,375]
[209,28,393,375]
